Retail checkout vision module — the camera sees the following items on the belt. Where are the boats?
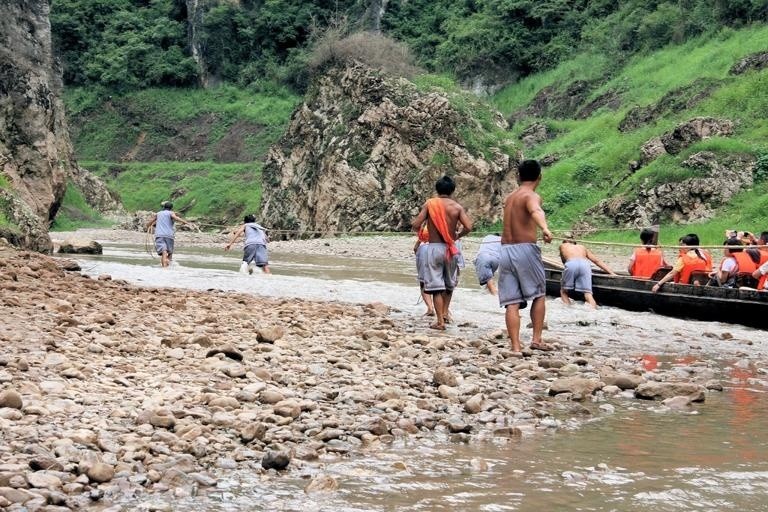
[541,254,767,333]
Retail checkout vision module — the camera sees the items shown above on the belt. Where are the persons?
[497,159,553,354]
[652,233,713,293]
[743,231,768,290]
[677,237,688,258]
[627,227,669,278]
[474,232,502,295]
[411,175,472,330]
[225,215,270,274]
[143,201,188,267]
[750,259,768,291]
[414,226,461,315]
[705,239,756,287]
[558,240,616,308]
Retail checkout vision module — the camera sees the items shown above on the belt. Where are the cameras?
[744,232,749,236]
[730,230,737,237]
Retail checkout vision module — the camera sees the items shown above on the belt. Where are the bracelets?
[657,282,661,288]
